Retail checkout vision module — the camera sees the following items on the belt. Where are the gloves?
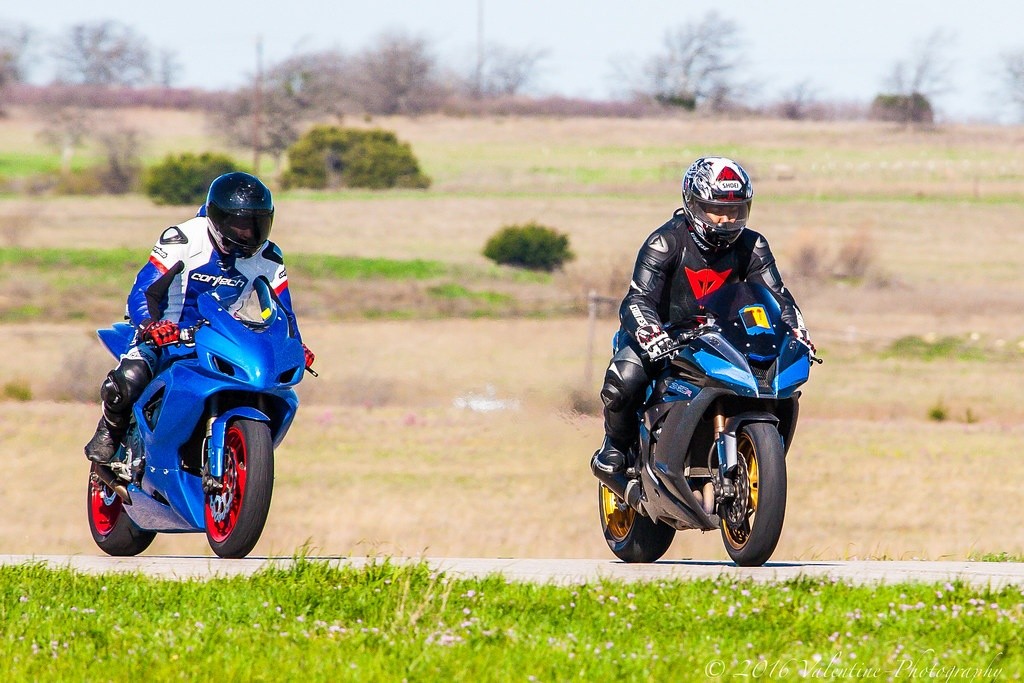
[793,328,816,366]
[301,342,315,369]
[137,318,181,348]
[635,323,679,360]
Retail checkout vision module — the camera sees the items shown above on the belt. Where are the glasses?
[226,215,267,231]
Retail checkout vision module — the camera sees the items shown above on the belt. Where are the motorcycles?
[590,282,823,567]
[86,279,318,562]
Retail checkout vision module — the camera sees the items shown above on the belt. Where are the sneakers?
[594,434,626,474]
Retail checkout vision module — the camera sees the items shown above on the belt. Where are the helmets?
[205,171,274,259]
[682,156,753,255]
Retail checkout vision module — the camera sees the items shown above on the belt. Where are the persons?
[595,157,817,473]
[85,171,315,463]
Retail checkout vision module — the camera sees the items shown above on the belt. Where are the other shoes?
[85,415,131,464]
